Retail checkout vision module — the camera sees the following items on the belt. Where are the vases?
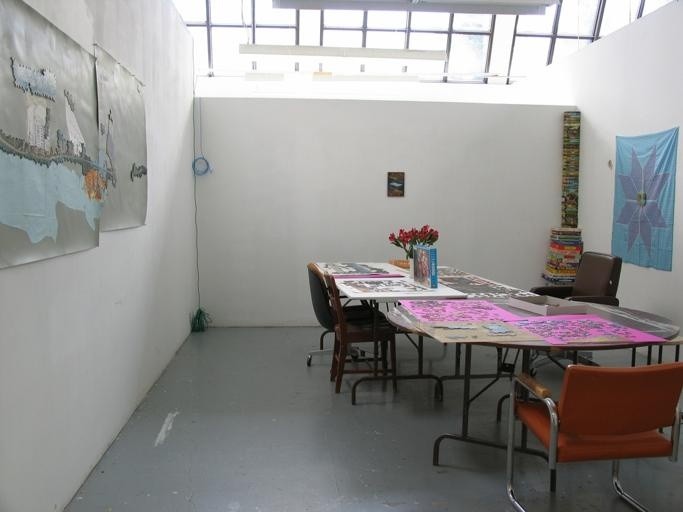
[408,257,414,276]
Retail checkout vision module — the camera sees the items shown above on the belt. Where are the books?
[541,227,583,284]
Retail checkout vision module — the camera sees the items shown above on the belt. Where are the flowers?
[389,225,438,255]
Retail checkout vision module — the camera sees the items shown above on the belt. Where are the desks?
[388,297,683,466]
[313,262,540,406]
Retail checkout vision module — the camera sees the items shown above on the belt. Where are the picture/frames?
[387,172,405,196]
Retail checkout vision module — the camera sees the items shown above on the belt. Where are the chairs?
[324,271,397,393]
[306,262,386,365]
[505,362,683,512]
[530,251,622,305]
